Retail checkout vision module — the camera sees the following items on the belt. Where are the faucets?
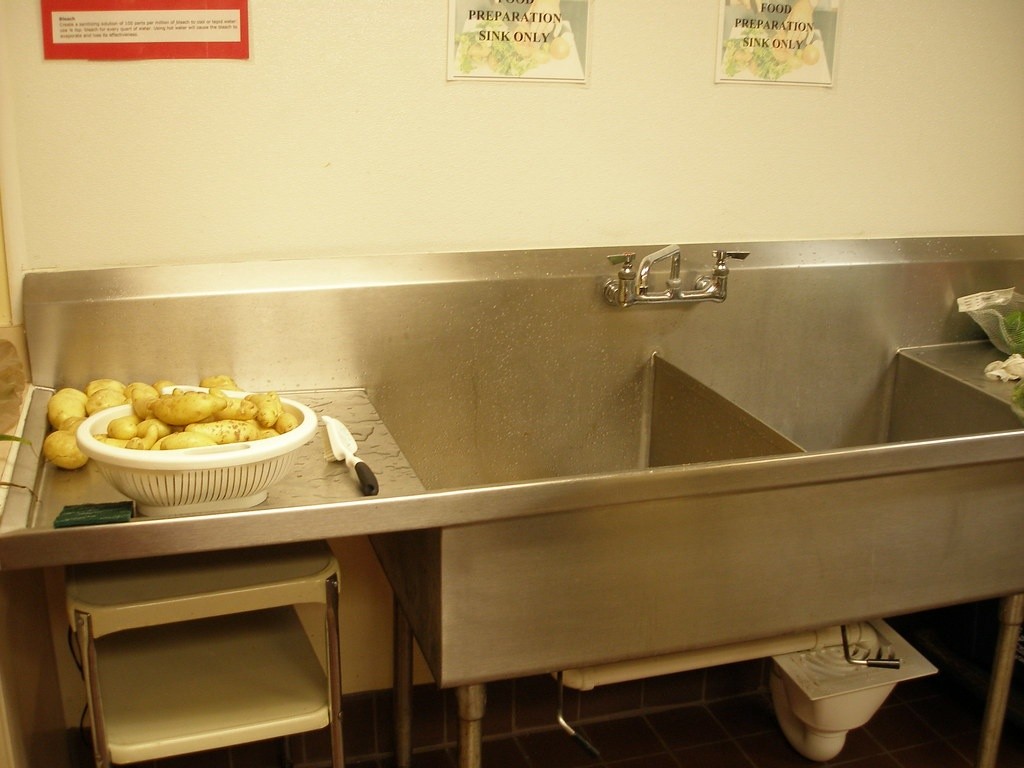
[636,243,687,310]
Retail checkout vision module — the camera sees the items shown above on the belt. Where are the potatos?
[42,373,299,468]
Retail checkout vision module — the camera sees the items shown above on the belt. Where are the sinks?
[676,342,1024,454]
[357,352,809,497]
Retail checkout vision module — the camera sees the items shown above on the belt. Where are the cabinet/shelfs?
[62,539,345,765]
